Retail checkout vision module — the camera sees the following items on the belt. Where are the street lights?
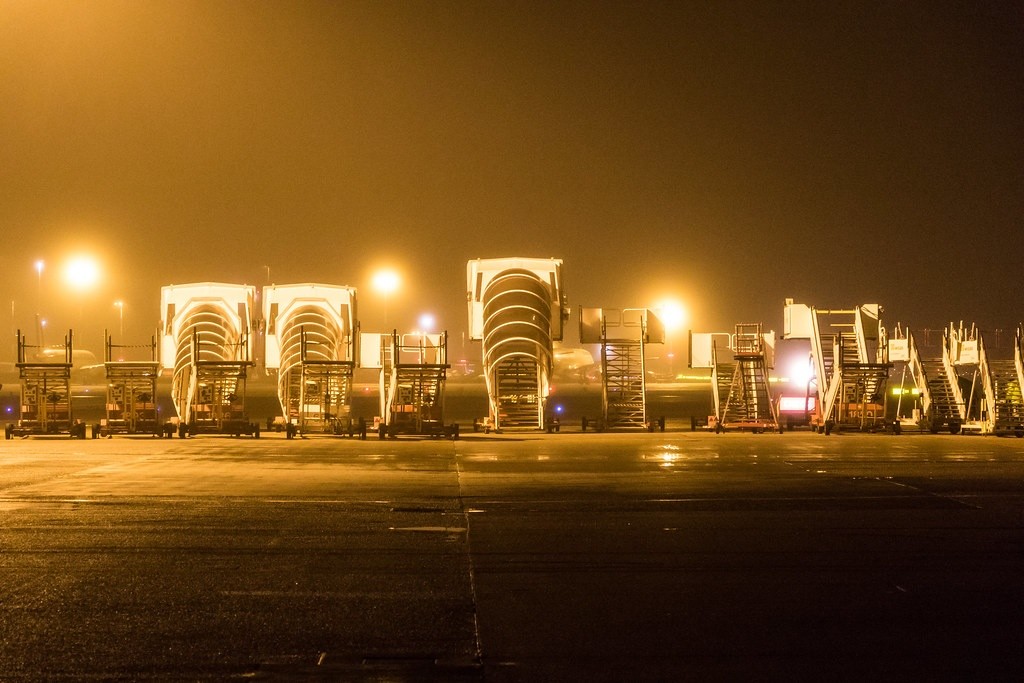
[35,260,43,354]
[374,268,400,334]
[114,300,125,363]
